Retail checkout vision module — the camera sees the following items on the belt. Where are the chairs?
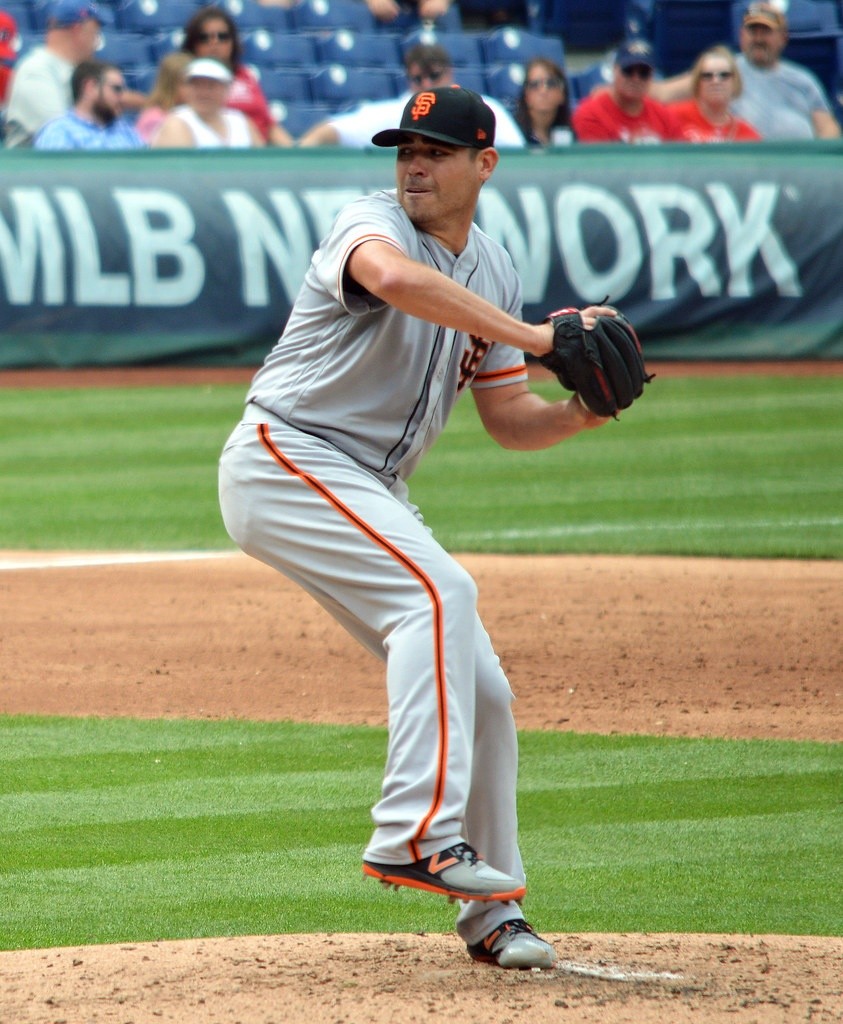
[0,0,843,149]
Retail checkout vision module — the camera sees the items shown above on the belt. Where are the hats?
[742,4,788,32]
[372,84,496,150]
[183,58,233,84]
[615,38,652,68]
[50,0,113,28]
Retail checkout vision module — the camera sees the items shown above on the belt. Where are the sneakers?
[362,842,528,905]
[467,920,557,974]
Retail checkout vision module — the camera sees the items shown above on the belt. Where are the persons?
[0,0,841,154]
[218,85,656,970]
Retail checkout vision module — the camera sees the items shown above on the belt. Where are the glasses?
[197,32,230,43]
[621,66,650,79]
[415,66,442,87]
[95,81,122,94]
[528,78,557,90]
[698,72,734,80]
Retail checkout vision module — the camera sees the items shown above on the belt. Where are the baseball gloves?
[535,302,650,416]
